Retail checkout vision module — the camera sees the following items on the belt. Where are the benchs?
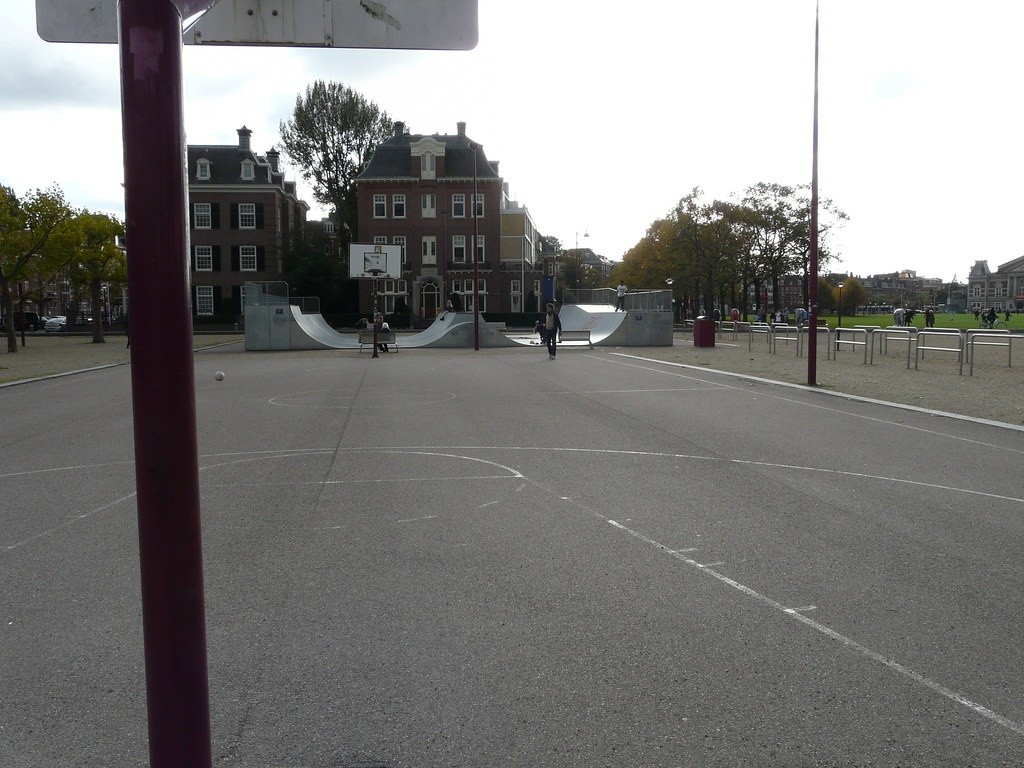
[359,328,399,354]
[486,322,506,332]
[559,331,594,351]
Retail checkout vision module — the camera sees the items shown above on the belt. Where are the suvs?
[0,311,39,332]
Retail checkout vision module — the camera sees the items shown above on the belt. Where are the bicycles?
[979,316,1007,329]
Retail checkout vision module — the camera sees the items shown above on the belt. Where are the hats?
[382,323,389,328]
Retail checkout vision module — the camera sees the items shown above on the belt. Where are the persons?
[981,307,999,324]
[439,300,461,321]
[356,317,370,329]
[377,316,388,352]
[753,307,789,326]
[534,302,561,360]
[124,319,130,348]
[1005,309,1010,321]
[615,280,628,312]
[903,309,910,327]
[924,308,934,328]
[975,310,979,320]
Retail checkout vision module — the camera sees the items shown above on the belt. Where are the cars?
[38,315,67,332]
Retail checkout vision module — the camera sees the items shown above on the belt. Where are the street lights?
[837,281,844,351]
[665,273,673,289]
[672,298,676,331]
[874,301,877,317]
[575,231,589,290]
[100,282,108,331]
[468,142,482,351]
[869,300,872,315]
[883,301,886,315]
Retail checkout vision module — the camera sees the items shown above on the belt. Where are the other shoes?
[379,346,389,353]
[549,353,556,360]
[615,308,625,312]
[440,317,444,321]
